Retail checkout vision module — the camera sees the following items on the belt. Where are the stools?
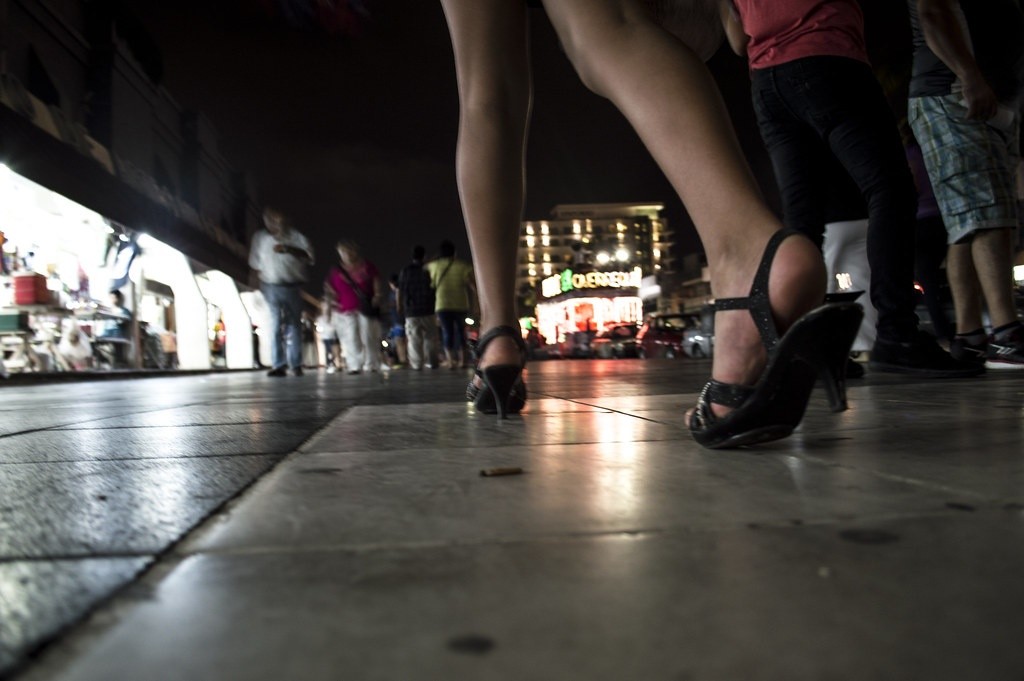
[0,329,37,376]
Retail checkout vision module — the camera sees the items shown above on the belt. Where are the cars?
[526,303,715,362]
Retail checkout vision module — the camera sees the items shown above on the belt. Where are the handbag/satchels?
[421,286,435,313]
[362,293,380,318]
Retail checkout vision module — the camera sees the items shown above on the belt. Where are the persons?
[715,0,1024,372]
[437,0,864,447]
[245,208,479,376]
[90,289,132,365]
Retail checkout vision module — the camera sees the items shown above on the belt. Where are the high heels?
[686,230,864,449]
[466,325,529,416]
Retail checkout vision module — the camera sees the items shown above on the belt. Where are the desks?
[0,305,75,375]
[70,311,131,371]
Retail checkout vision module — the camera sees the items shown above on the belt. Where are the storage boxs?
[0,308,29,332]
[13,271,48,304]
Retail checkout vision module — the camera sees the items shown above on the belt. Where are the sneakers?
[867,326,986,374]
[841,355,864,378]
[953,334,989,373]
[984,328,1024,369]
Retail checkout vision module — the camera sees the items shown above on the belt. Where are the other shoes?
[268,369,286,377]
[293,366,304,378]
